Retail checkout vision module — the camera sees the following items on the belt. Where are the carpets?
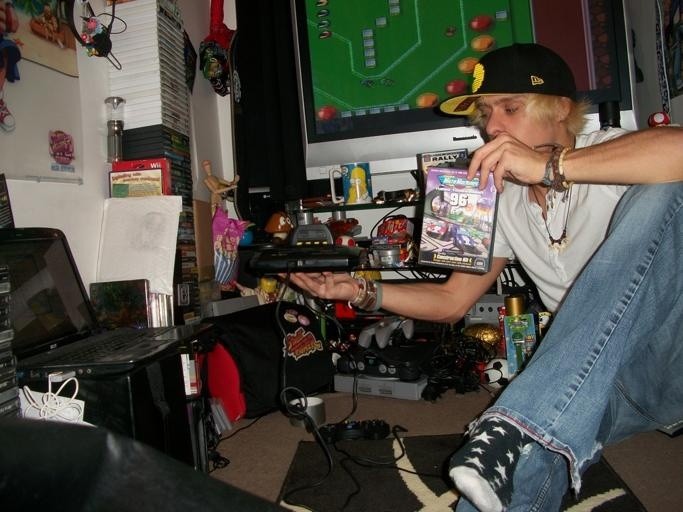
[272,434,645,512]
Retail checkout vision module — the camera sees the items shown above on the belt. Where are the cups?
[329,160,373,206]
[239,230,252,245]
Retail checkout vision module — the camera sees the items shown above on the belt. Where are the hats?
[438,43,576,116]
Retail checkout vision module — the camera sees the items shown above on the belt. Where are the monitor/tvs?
[289,1,639,201]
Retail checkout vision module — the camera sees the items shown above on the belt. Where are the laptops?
[0,227,214,380]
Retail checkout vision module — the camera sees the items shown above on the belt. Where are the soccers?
[484,358,510,389]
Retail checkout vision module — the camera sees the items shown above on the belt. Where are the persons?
[40,3,58,32]
[275,41,683,512]
[0,0,21,131]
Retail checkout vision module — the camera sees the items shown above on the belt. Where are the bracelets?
[352,276,384,312]
[542,145,573,199]
